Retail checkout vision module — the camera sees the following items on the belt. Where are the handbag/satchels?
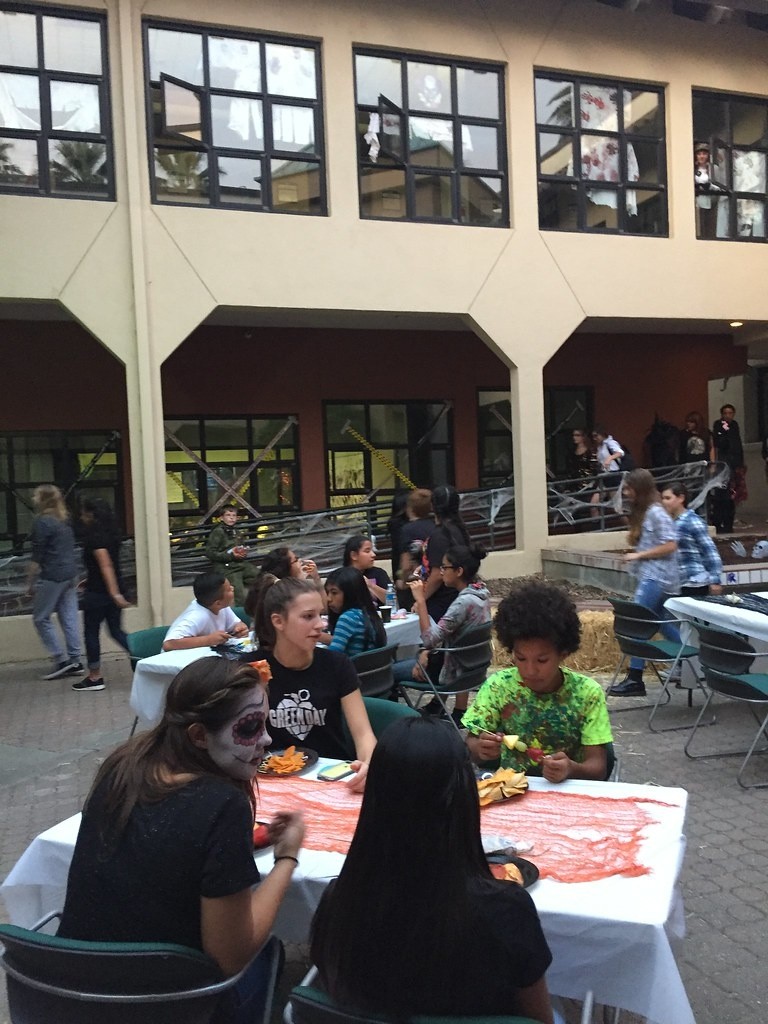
[731,468,747,501]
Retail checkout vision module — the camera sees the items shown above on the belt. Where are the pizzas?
[246,659,273,684]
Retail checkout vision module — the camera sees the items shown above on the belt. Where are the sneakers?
[72,678,105,691]
[658,664,683,681]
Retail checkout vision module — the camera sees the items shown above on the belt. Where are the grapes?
[514,741,528,752]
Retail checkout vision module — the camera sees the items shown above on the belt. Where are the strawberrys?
[526,747,544,761]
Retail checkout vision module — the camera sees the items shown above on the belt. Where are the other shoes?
[449,710,467,729]
[420,705,444,717]
[732,519,753,529]
[66,664,85,676]
[444,714,455,721]
[41,660,72,679]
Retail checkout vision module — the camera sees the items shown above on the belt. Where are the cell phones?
[318,761,356,782]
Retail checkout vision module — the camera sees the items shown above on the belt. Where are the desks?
[675,591,768,708]
[129,610,431,737]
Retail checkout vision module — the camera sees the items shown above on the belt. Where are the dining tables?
[38,750,688,1024]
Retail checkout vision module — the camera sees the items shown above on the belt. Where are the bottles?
[385,583,398,615]
[227,544,250,555]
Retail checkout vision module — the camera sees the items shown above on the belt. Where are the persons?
[660,482,723,597]
[159,485,490,729]
[695,142,720,240]
[310,715,560,1024]
[460,579,614,783]
[71,495,139,691]
[28,484,86,678]
[606,468,683,697]
[50,656,306,979]
[674,403,768,534]
[571,425,634,525]
[240,573,378,794]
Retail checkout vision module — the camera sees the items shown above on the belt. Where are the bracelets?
[274,855,299,865]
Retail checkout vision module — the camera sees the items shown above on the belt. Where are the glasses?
[440,564,459,571]
[289,556,298,565]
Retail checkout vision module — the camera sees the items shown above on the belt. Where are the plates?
[485,853,539,888]
[256,747,318,776]
[251,822,274,853]
[475,769,529,804]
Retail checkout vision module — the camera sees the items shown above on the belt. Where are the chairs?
[283,962,596,1024]
[604,597,717,732]
[127,622,620,781]
[0,909,284,1024]
[684,622,768,789]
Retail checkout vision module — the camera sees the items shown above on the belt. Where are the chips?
[267,745,306,774]
[477,766,528,806]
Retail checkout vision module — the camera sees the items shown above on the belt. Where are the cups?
[378,606,392,623]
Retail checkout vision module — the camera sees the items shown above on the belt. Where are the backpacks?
[606,442,634,471]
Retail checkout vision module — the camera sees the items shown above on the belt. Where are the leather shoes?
[607,680,646,696]
